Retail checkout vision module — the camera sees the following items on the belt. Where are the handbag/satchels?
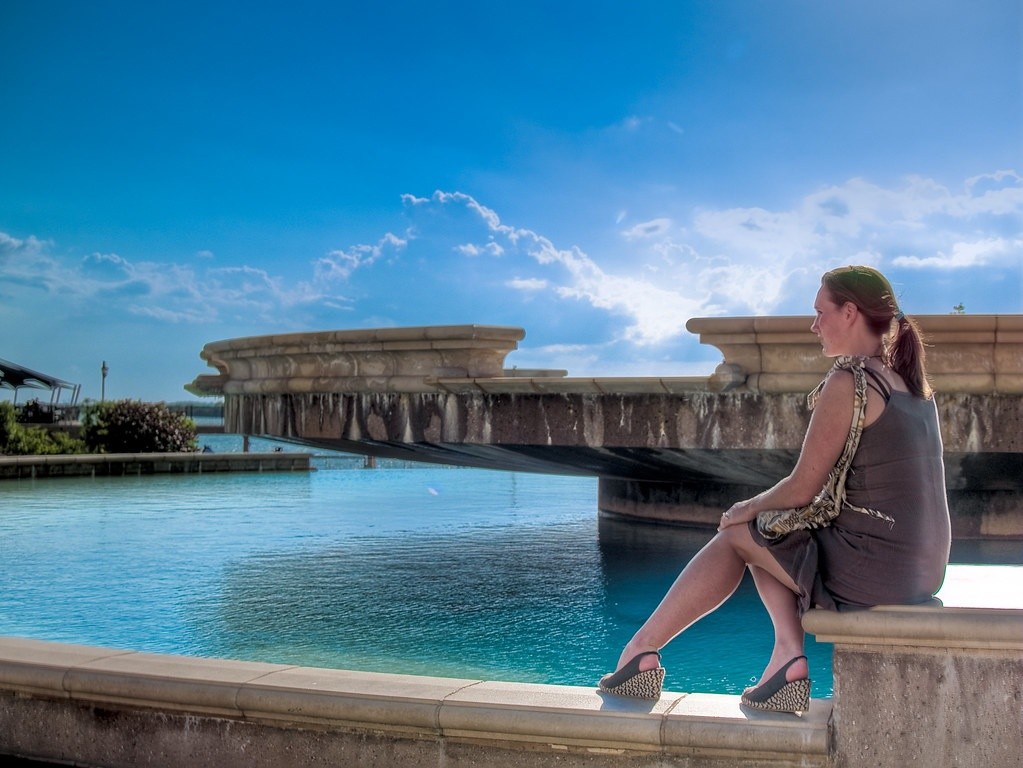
[756,354,869,540]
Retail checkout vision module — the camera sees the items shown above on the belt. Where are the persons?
[597,265,952,714]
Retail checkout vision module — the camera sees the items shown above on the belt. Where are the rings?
[722,512,728,521]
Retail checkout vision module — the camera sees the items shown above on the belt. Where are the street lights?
[101,361,109,402]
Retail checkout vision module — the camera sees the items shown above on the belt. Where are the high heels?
[599,651,665,699]
[741,655,811,712]
